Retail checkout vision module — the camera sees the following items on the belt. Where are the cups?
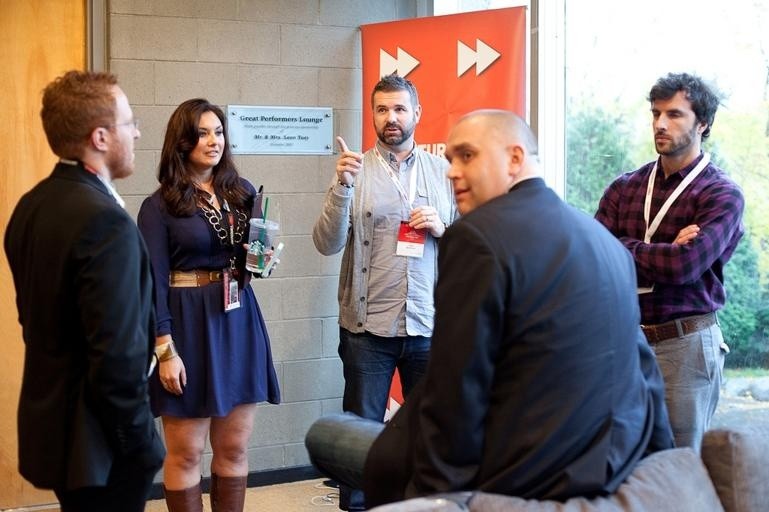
[245,217,281,275]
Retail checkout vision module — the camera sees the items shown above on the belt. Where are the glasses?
[102,115,142,130]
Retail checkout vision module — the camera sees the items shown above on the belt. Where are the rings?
[163,381,168,389]
[426,215,429,222]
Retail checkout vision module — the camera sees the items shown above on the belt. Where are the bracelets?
[336,179,356,188]
[154,339,178,363]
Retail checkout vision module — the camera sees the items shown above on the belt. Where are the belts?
[639,309,721,344]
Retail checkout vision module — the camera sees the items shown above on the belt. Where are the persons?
[1,69,172,512]
[134,98,280,512]
[303,106,679,502]
[310,70,463,512]
[593,72,749,457]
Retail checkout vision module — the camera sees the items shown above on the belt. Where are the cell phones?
[261,243,285,278]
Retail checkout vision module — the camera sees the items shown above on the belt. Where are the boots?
[164,474,206,512]
[210,473,248,511]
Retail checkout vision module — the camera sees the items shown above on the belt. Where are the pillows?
[365,422,769,512]
